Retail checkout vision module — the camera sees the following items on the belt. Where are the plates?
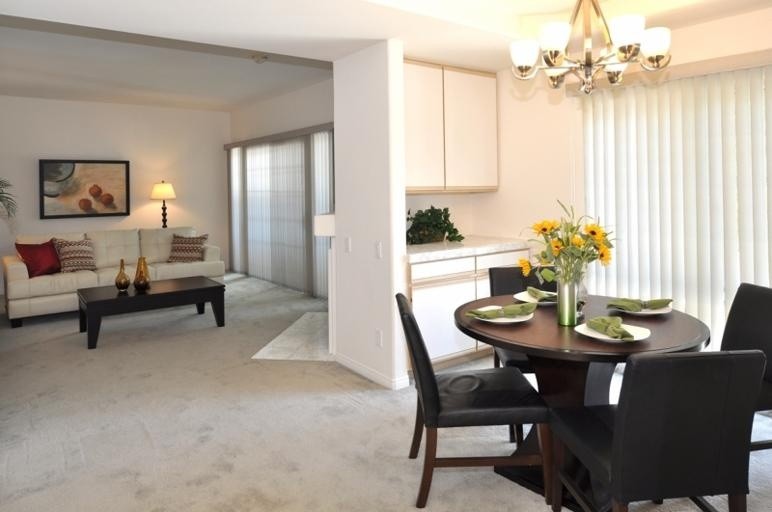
[473,305,535,325]
[621,301,672,316]
[574,322,651,343]
[512,290,557,307]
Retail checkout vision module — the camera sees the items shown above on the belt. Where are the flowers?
[517,199,622,284]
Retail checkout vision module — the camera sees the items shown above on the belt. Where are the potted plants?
[406,203,465,246]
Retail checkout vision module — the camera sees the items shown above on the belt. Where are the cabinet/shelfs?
[313,213,336,354]
[408,248,531,378]
[404,57,499,195]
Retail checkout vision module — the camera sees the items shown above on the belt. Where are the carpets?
[251,311,335,362]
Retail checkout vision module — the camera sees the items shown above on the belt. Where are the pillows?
[14,236,62,278]
[52,236,98,273]
[166,232,210,263]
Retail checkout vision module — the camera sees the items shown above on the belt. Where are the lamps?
[150,180,176,228]
[508,0,673,96]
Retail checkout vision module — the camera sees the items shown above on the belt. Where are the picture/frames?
[39,158,130,220]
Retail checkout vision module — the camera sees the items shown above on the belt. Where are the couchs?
[2,226,227,328]
